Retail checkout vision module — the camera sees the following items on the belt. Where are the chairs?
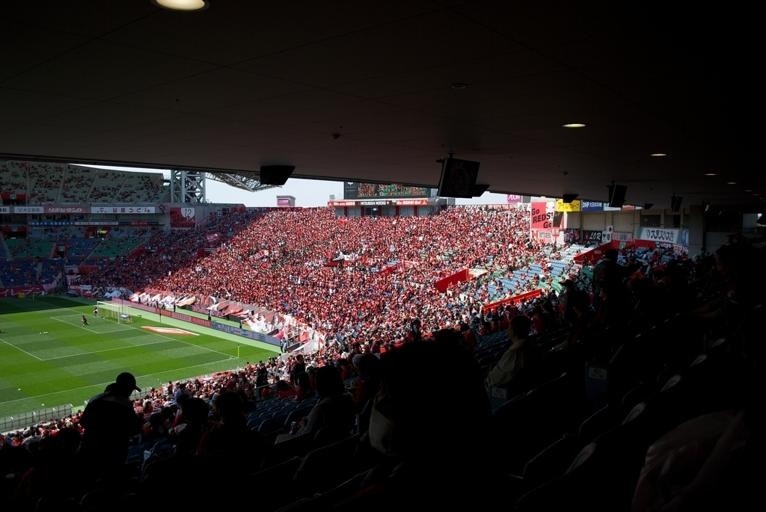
[138,246,679,512]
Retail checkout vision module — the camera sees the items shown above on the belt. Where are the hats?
[116,372,142,392]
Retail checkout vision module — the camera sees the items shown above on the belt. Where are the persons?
[1,204,766,511]
